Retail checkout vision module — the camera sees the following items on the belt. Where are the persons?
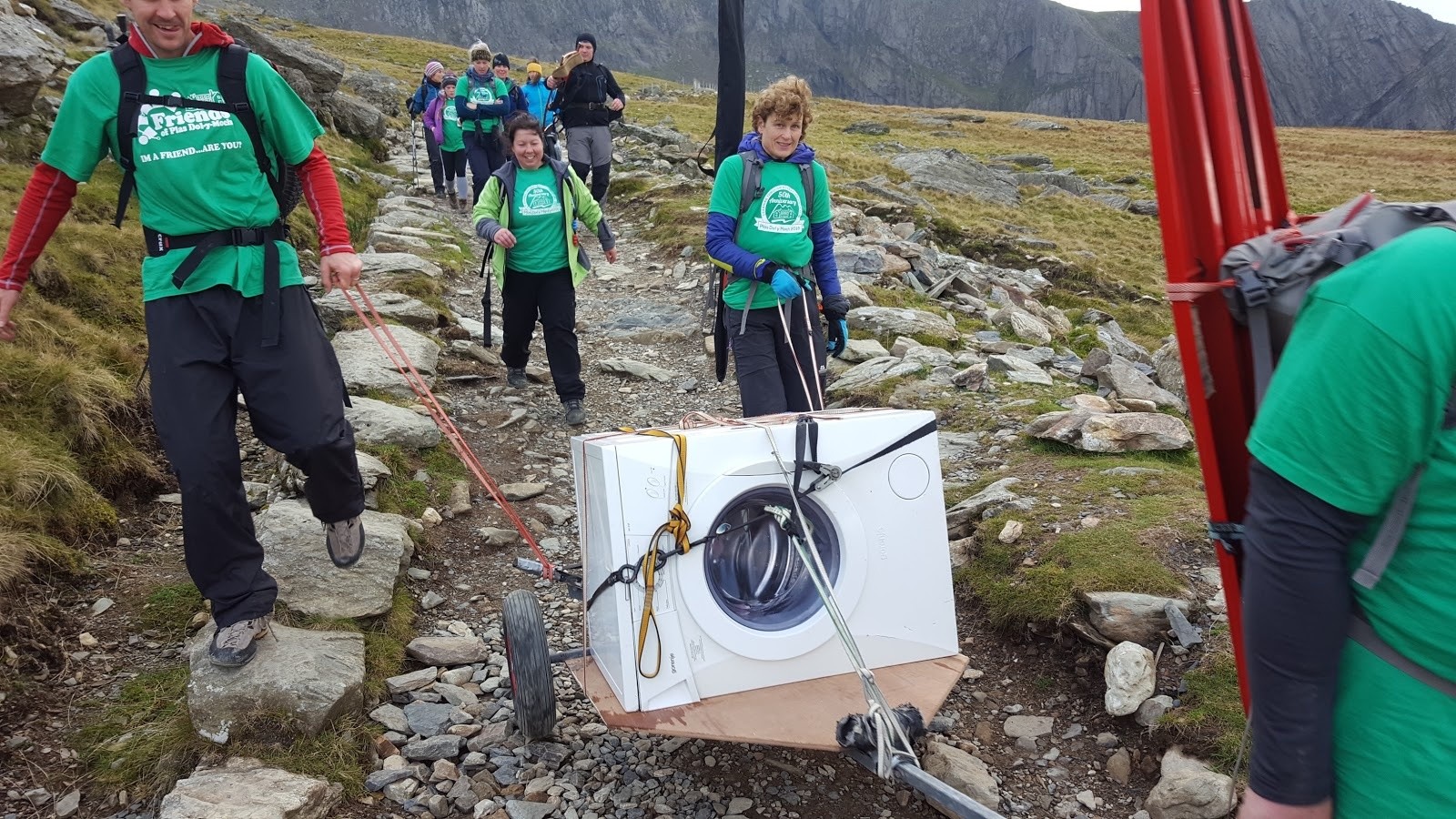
[405,41,556,191]
[472,114,618,426]
[1236,220,1456,819]
[708,74,850,416]
[0,0,365,668]
[545,34,626,231]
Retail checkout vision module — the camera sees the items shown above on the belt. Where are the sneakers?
[447,190,459,209]
[506,364,529,389]
[459,198,468,214]
[563,399,586,424]
[208,603,280,667]
[321,513,366,568]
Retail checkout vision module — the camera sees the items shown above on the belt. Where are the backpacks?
[1223,189,1456,694]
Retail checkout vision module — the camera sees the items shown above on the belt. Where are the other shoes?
[434,187,446,202]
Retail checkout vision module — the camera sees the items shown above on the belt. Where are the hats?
[576,33,597,52]
[492,53,510,70]
[426,61,445,78]
[441,70,458,88]
[527,62,542,75]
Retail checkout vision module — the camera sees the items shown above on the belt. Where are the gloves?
[760,261,812,301]
[406,97,413,109]
[826,318,850,359]
[489,125,499,151]
[473,119,486,144]
